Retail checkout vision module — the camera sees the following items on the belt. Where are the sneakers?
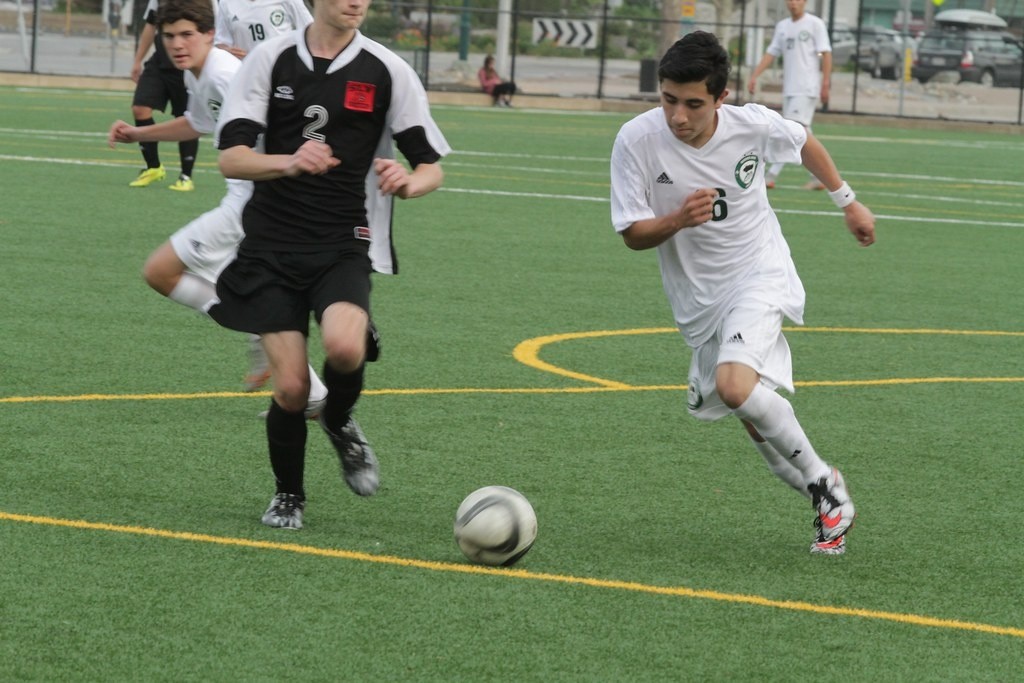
[810,497,846,554]
[258,396,325,418]
[170,178,194,191]
[808,464,857,543]
[129,164,166,186]
[242,335,273,392]
[263,491,304,528]
[318,400,379,495]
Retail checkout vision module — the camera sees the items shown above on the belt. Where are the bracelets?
[829,180,855,207]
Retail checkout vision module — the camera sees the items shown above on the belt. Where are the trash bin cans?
[638,58,658,93]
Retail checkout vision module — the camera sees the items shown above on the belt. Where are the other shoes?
[765,176,776,188]
[803,177,825,189]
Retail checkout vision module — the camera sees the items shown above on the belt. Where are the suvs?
[913,9,1024,87]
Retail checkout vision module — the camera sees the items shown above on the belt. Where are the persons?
[479,55,516,108]
[609,30,876,553]
[212,1,452,528]
[212,1,313,60]
[107,0,329,422]
[748,0,833,190]
[128,0,199,191]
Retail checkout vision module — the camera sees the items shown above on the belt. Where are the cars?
[852,26,904,80]
[817,22,858,70]
[900,33,917,71]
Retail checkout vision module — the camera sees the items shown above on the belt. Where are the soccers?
[453,483,540,568]
